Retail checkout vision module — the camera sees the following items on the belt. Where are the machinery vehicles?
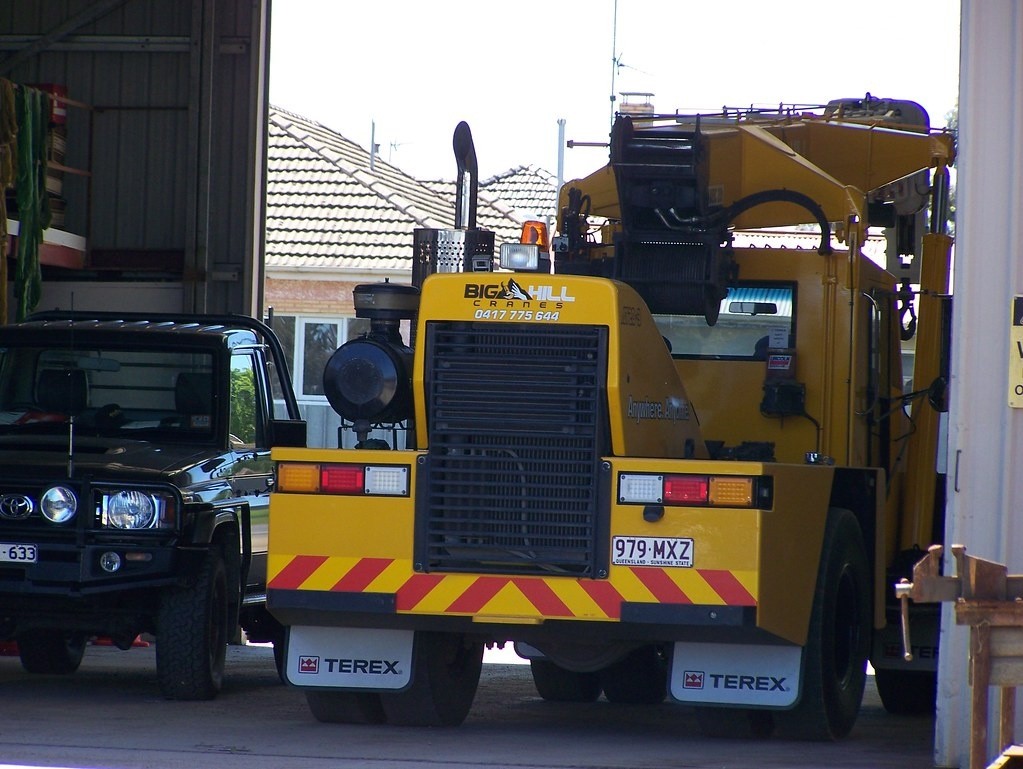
[259,95,954,744]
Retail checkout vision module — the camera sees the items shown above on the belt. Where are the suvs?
[2,307,308,706]
